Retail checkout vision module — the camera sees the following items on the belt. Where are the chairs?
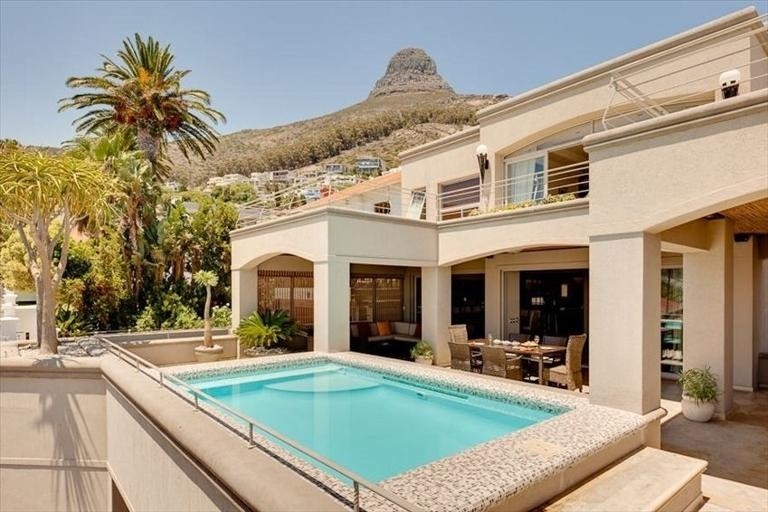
[448,325,588,392]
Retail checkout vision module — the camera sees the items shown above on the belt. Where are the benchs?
[541,448,714,512]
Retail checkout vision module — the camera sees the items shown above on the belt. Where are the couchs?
[296,321,420,351]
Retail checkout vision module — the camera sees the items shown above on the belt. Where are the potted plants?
[409,340,433,365]
[194,270,222,362]
[233,306,294,357]
[680,369,718,424]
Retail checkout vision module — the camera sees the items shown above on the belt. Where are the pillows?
[377,322,392,336]
